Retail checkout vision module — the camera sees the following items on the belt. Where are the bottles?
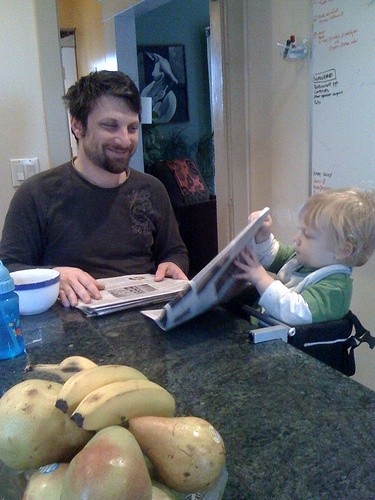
[0,260,25,360]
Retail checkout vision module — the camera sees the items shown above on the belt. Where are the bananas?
[23,355,176,430]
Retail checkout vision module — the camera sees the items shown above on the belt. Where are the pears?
[0,379,91,469]
[23,416,226,500]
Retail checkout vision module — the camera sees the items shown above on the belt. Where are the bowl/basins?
[7,268,60,315]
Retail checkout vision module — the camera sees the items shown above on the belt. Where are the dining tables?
[1,278,375,500]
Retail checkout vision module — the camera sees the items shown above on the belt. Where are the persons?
[232,189,375,341]
[0,69,190,308]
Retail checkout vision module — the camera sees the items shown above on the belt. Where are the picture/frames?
[136,44,189,125]
